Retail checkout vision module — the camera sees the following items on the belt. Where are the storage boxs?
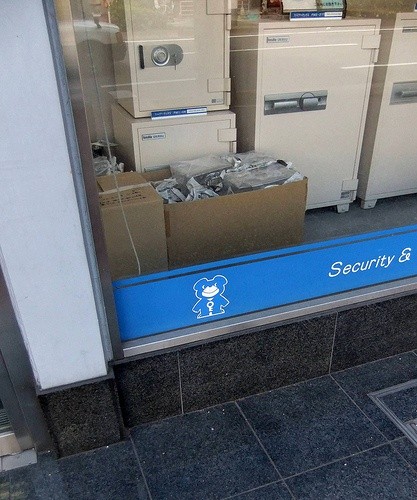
[96,168,169,281]
[143,154,309,271]
